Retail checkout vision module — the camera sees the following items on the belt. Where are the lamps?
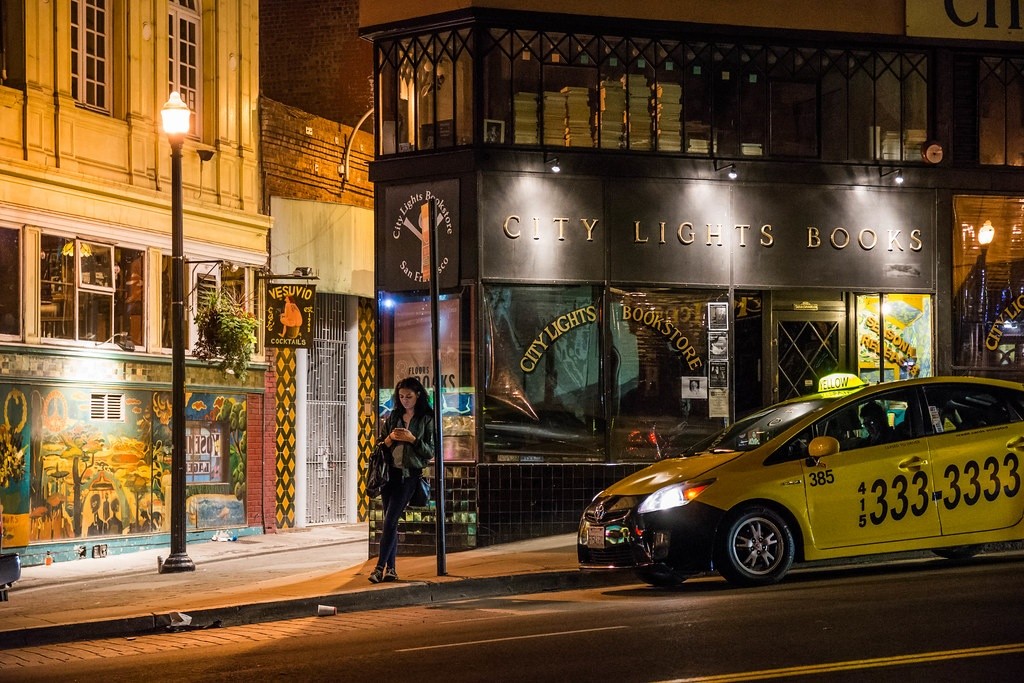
[545,156,561,173]
[89,330,135,353]
[62,240,92,258]
[196,149,214,162]
[880,168,904,184]
[715,163,738,180]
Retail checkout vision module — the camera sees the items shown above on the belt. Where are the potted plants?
[192,283,265,382]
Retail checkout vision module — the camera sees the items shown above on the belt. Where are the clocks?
[917,141,946,164]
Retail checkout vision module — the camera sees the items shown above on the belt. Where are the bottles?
[45,550,52,566]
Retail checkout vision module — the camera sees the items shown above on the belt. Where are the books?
[882,128,927,161]
[511,73,763,155]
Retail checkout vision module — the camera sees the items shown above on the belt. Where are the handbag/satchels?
[365,442,390,499]
[409,476,431,507]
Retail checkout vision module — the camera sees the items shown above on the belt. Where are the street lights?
[976,218,996,368]
[159,91,197,573]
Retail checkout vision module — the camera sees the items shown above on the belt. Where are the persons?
[846,402,904,450]
[978,402,1020,427]
[368,377,435,583]
[691,382,698,391]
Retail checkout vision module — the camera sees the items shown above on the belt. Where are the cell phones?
[395,427,405,431]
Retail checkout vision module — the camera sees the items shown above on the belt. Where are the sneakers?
[382,566,398,582]
[368,567,383,584]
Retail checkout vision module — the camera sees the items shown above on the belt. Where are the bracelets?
[389,435,394,442]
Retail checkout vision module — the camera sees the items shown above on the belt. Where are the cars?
[574,372,1023,587]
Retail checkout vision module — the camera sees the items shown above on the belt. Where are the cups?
[318,604,338,617]
